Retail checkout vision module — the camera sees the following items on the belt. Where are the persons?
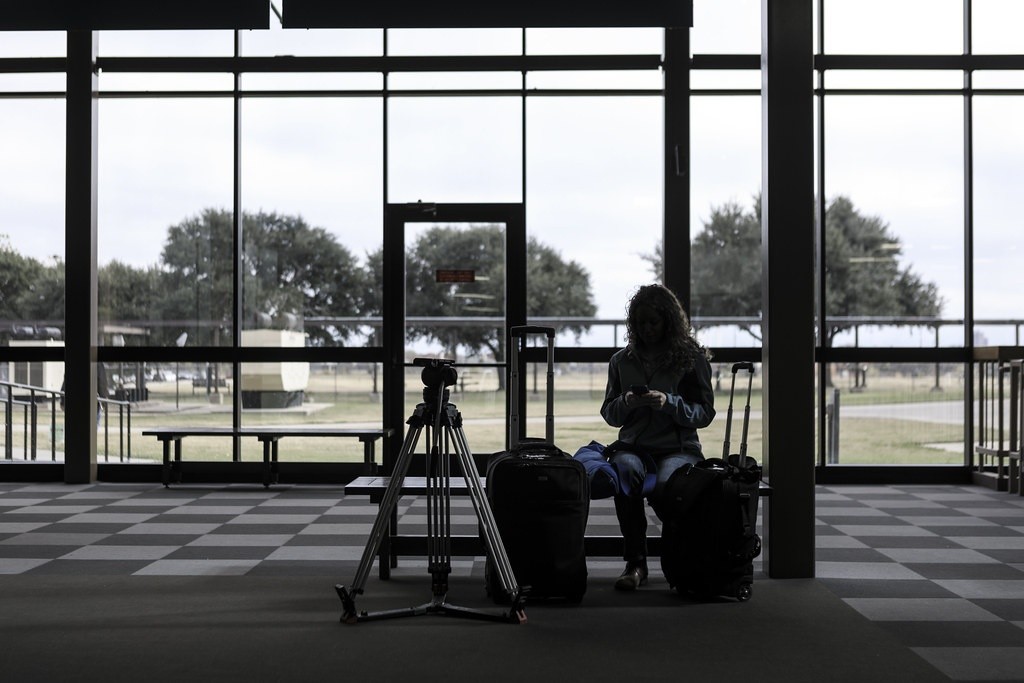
[600,285,717,591]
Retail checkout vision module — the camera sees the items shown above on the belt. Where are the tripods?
[335,359,531,624]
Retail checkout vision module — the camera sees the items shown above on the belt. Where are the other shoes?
[614,561,647,591]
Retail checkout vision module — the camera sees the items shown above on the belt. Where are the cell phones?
[630,384,650,398]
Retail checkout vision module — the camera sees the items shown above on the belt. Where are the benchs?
[344,476,773,585]
[142,427,394,489]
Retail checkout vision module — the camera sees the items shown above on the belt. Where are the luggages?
[660,364,762,601]
[478,327,589,608]
